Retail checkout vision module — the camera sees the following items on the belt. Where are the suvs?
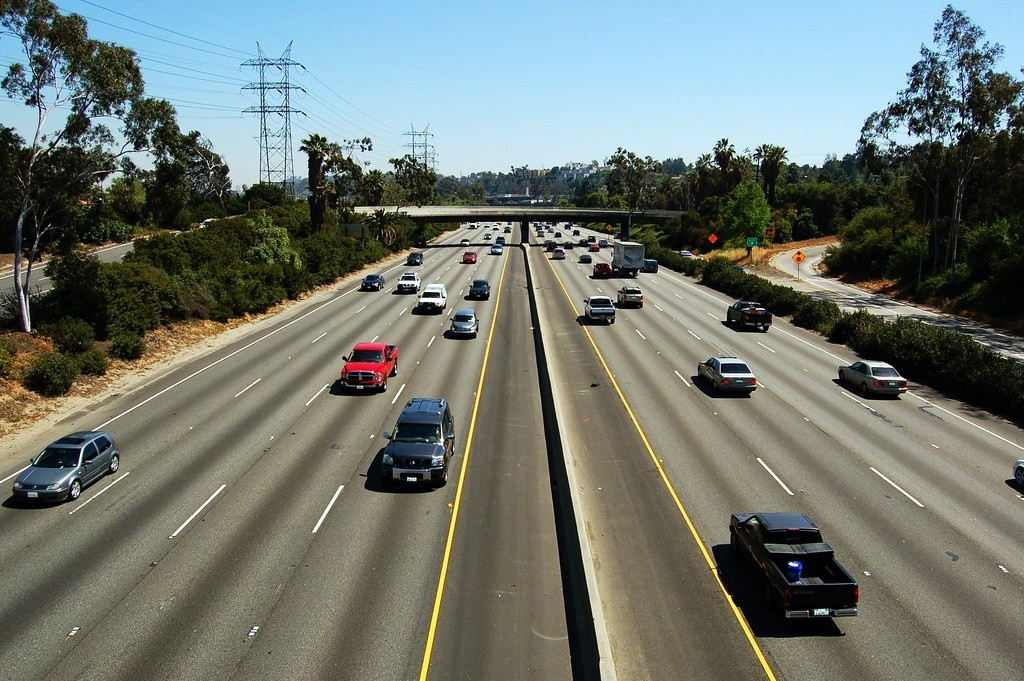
[381,396,456,487]
[12,431,120,505]
[640,259,659,273]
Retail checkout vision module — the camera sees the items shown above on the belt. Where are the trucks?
[417,284,448,314]
[611,240,646,278]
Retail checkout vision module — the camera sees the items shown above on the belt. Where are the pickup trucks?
[727,301,773,331]
[340,341,398,395]
[729,512,860,619]
[583,296,616,324]
[396,273,421,293]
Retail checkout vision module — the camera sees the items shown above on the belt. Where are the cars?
[1012,459,1024,489]
[592,262,612,280]
[616,285,644,308]
[460,222,512,247]
[469,278,493,301]
[406,252,425,266]
[490,243,504,255]
[680,250,692,260]
[837,359,908,397]
[462,250,477,264]
[361,273,386,291]
[578,254,592,263]
[697,355,758,393]
[449,309,480,340]
[532,222,609,252]
[552,248,566,260]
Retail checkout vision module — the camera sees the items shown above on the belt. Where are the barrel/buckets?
[787,561,802,581]
